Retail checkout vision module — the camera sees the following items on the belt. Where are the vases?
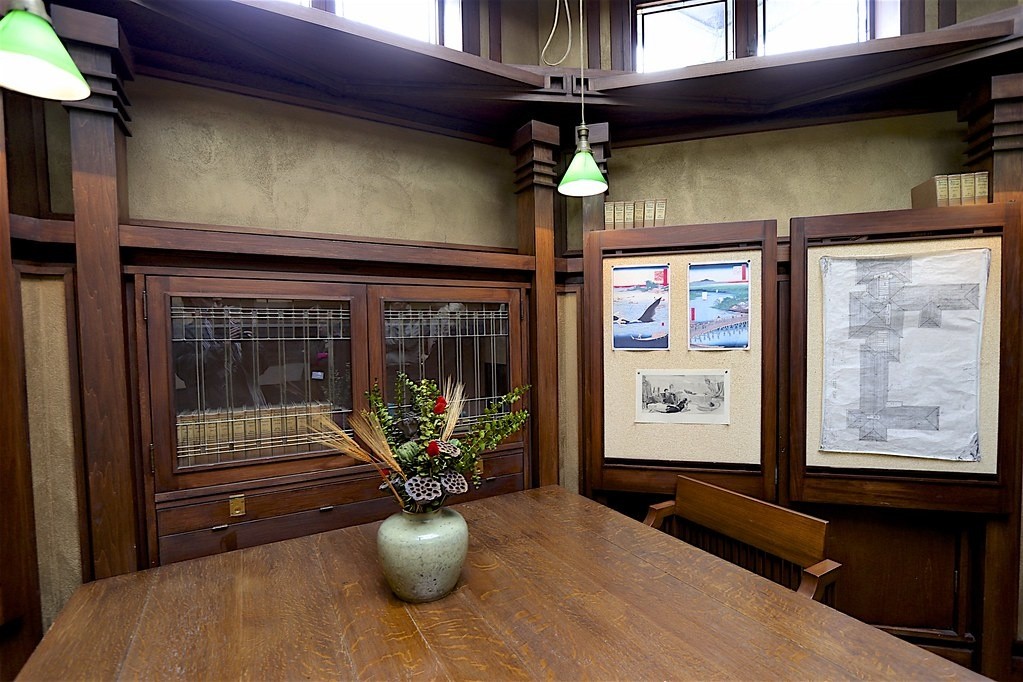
[377,502,472,601]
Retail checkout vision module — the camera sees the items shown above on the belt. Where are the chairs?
[642,474,842,601]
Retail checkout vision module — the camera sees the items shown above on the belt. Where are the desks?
[14,483,996,682]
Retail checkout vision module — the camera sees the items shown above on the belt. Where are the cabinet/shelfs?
[126,257,532,575]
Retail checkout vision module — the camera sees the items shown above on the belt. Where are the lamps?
[559,1,608,199]
[0,0,92,101]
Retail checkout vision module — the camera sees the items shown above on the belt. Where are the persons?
[183,298,266,412]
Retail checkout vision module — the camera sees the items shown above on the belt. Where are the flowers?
[306,366,526,509]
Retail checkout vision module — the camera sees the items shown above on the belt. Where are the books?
[173,399,344,468]
